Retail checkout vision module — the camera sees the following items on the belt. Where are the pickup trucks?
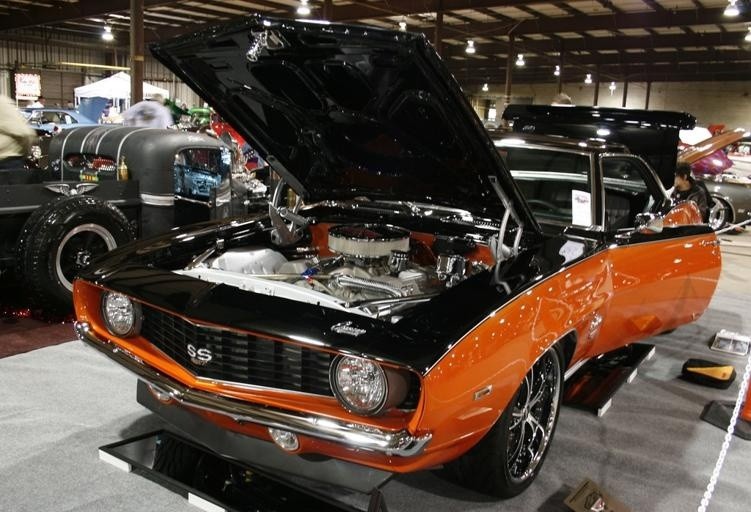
[0,124,249,330]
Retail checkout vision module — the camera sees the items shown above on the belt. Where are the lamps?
[722,0,742,18]
[514,52,526,67]
[554,64,560,76]
[295,0,310,16]
[100,21,114,42]
[463,38,475,55]
[582,72,594,84]
[481,83,489,91]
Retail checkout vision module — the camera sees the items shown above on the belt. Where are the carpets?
[0,207,750,511]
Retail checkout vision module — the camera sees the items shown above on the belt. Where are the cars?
[677,124,750,240]
[22,98,110,138]
[68,13,726,502]
[167,101,269,203]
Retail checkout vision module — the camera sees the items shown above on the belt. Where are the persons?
[101,93,188,130]
[0,94,45,168]
[551,92,571,105]
[675,160,710,224]
